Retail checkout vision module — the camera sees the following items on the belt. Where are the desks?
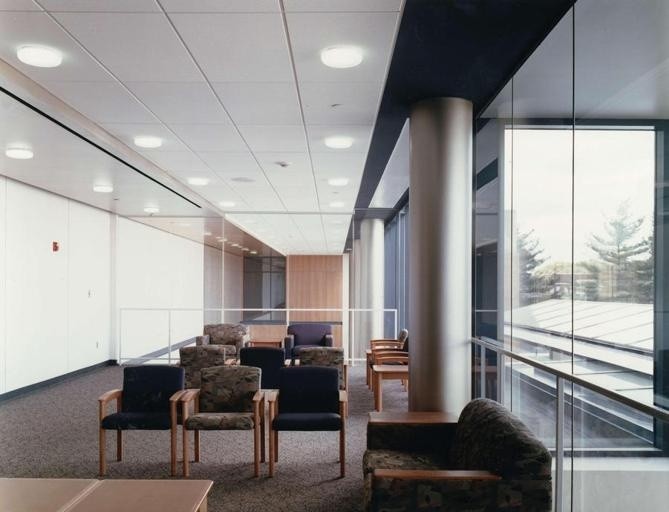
[371,365,410,409]
[0,476,216,512]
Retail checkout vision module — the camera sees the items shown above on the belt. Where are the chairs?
[366,328,410,390]
[363,398,556,511]
[97,322,347,480]
